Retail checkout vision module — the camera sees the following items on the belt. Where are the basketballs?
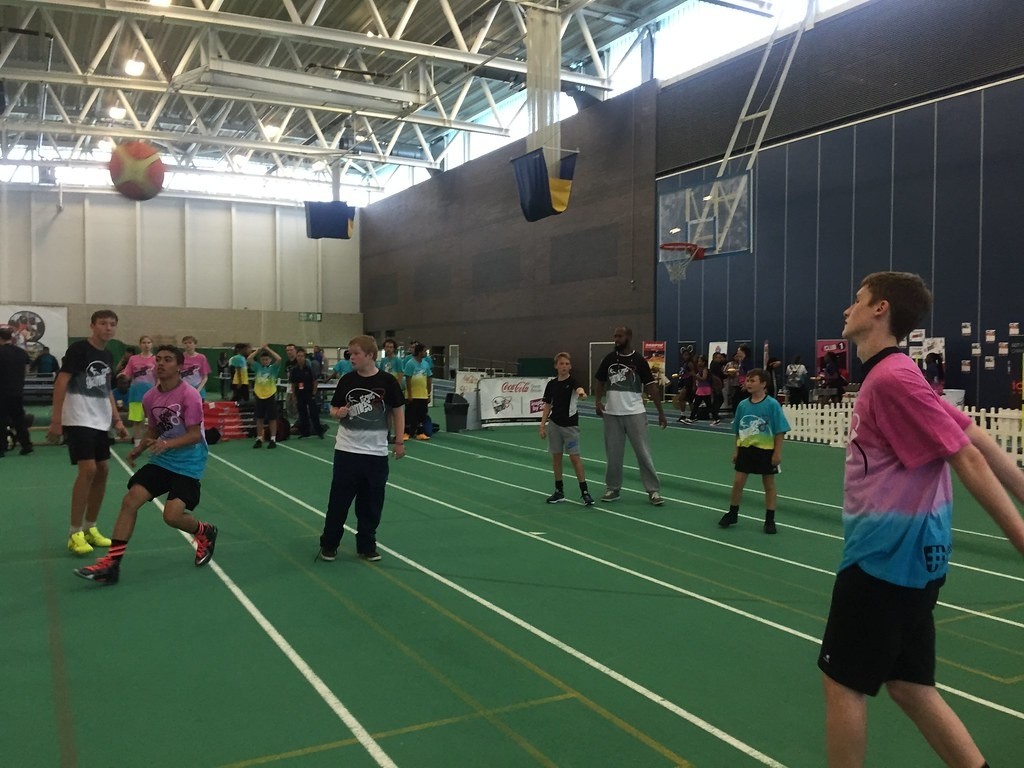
[110,141,164,200]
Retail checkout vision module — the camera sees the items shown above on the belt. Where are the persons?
[818,270,1024,768]
[326,340,434,441]
[717,369,791,534]
[921,350,942,384]
[0,326,35,458]
[30,347,60,377]
[73,345,218,583]
[594,325,669,505]
[674,344,845,427]
[539,352,595,506]
[115,335,212,448]
[318,335,407,562]
[44,309,129,553]
[218,342,329,449]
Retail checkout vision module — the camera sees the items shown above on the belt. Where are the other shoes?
[268,441,276,449]
[386,434,397,443]
[19,443,33,455]
[710,420,720,427]
[253,440,260,448]
[677,416,693,423]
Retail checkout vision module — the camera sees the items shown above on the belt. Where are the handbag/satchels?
[696,386,712,396]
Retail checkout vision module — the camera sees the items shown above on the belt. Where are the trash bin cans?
[444,393,470,433]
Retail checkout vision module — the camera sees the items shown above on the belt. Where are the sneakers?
[546,489,565,503]
[648,490,665,505]
[74,559,120,584]
[84,527,113,547]
[193,522,218,567]
[718,512,738,527]
[600,488,620,502]
[358,549,381,561]
[764,521,776,534]
[403,433,409,441]
[314,543,338,561]
[580,491,595,505]
[67,530,93,555]
[416,433,431,440]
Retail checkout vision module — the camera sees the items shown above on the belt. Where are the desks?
[276,383,337,415]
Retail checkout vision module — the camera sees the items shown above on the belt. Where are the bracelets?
[596,401,601,405]
[428,394,431,397]
[312,395,315,397]
[161,440,169,448]
[396,441,404,445]
[114,419,122,425]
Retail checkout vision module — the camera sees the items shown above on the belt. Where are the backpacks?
[701,368,722,393]
